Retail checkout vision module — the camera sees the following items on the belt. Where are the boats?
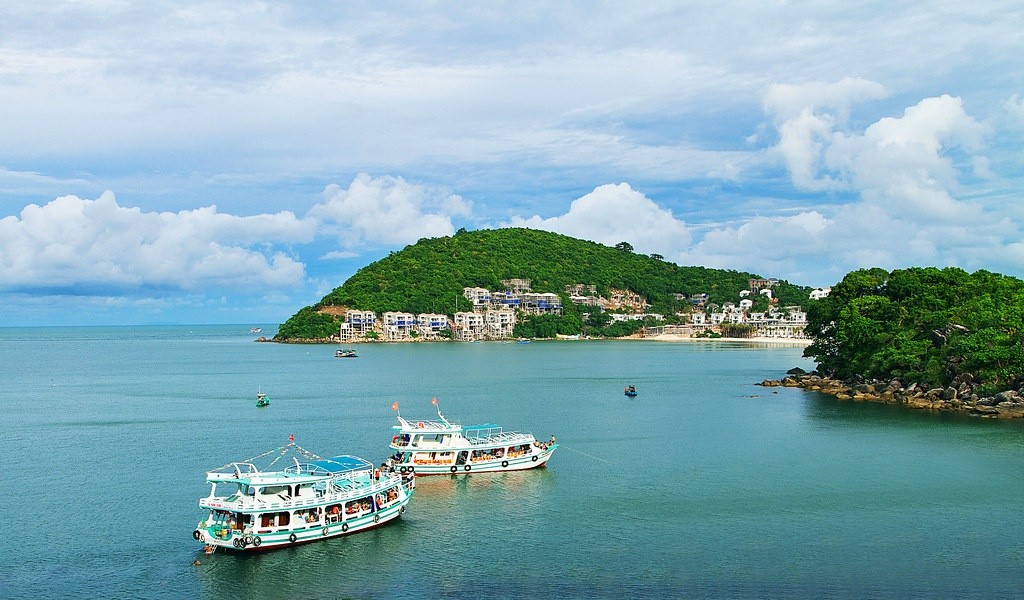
[387,411,559,478]
[192,433,416,553]
[255,392,271,407]
[250,328,262,333]
[334,348,358,357]
[624,384,638,396]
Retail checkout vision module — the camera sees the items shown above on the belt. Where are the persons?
[458,444,530,465]
[193,559,201,566]
[370,468,381,485]
[391,434,410,446]
[534,435,559,451]
[202,487,413,541]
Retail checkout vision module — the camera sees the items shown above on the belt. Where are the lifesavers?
[342,523,349,532]
[502,461,508,467]
[245,536,253,544]
[323,527,329,536]
[193,531,200,539]
[289,534,297,542]
[532,456,538,461]
[400,466,414,473]
[374,515,380,523]
[450,465,471,473]
[199,533,207,543]
[238,538,246,548]
[233,538,240,547]
[399,506,406,514]
[253,536,261,546]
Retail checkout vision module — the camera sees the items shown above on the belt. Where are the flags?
[432,397,437,406]
[391,401,401,408]
